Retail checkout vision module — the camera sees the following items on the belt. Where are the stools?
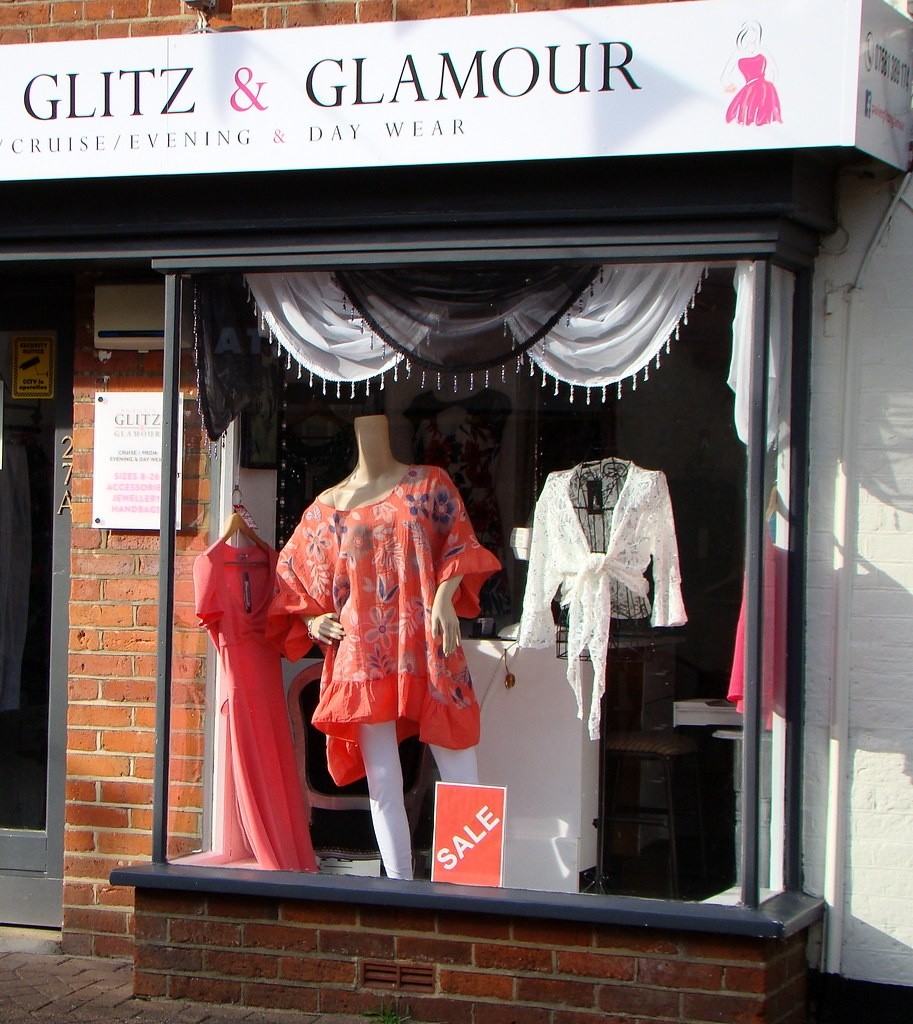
[605,730,706,900]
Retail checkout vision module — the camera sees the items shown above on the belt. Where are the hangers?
[277,400,353,439]
[195,488,279,564]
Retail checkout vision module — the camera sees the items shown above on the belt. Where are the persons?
[278,409,502,888]
